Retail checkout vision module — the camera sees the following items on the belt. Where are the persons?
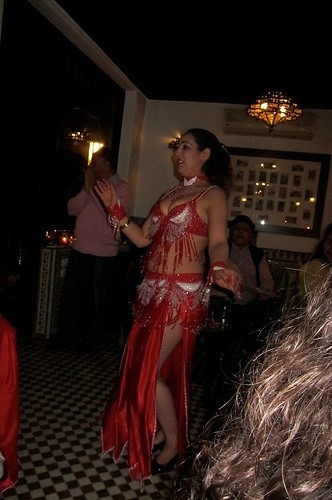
[183,264,332,500]
[227,215,275,375]
[299,224,332,306]
[46,147,128,350]
[94,128,244,480]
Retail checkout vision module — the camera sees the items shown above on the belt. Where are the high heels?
[149,452,178,473]
[152,441,166,453]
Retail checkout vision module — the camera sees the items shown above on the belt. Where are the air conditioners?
[223,109,317,141]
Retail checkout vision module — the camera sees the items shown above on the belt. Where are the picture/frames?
[227,146,331,239]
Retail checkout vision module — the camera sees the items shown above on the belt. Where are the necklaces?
[184,176,208,186]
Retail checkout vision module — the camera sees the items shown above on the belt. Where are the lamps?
[246,86,303,133]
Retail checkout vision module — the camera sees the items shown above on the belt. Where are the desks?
[0,314,18,493]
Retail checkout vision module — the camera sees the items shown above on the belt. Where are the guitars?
[208,255,285,303]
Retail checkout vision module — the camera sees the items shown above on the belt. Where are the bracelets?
[211,260,227,270]
[120,218,131,229]
[106,203,126,220]
